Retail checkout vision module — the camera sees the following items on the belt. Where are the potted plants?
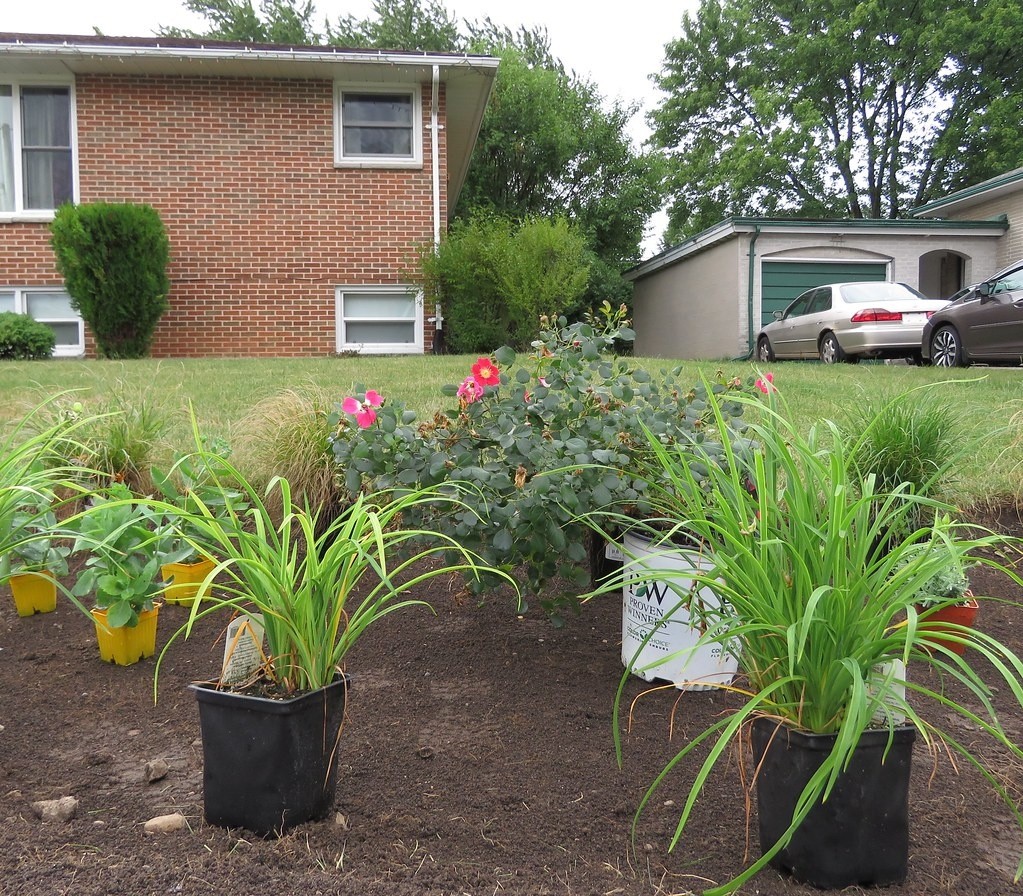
[253,401,350,559]
[845,391,954,555]
[887,542,978,655]
[9,460,64,617]
[69,478,195,667]
[572,373,1023,896]
[106,415,520,838]
[137,436,249,607]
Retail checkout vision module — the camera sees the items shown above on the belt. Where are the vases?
[584,513,685,592]
[612,522,745,692]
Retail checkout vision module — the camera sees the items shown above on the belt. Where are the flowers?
[322,284,780,585]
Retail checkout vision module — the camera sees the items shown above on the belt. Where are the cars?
[921,259,1023,365]
[758,281,954,367]
